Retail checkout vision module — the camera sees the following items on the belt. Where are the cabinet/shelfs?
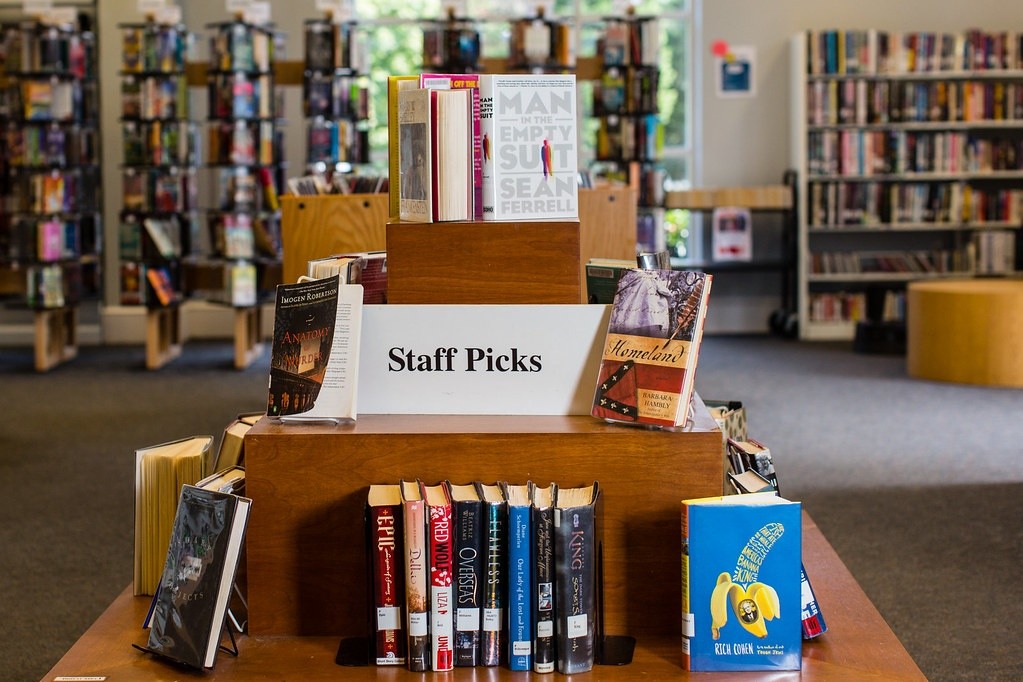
[1,5,665,341]
[787,26,1023,345]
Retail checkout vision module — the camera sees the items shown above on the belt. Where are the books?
[387,74,579,223]
[584,255,712,428]
[807,28,1023,322]
[679,405,826,671]
[0,20,283,311]
[133,411,267,671]
[423,22,568,72]
[592,17,752,265]
[367,479,606,674]
[267,252,387,422]
[288,20,388,194]
